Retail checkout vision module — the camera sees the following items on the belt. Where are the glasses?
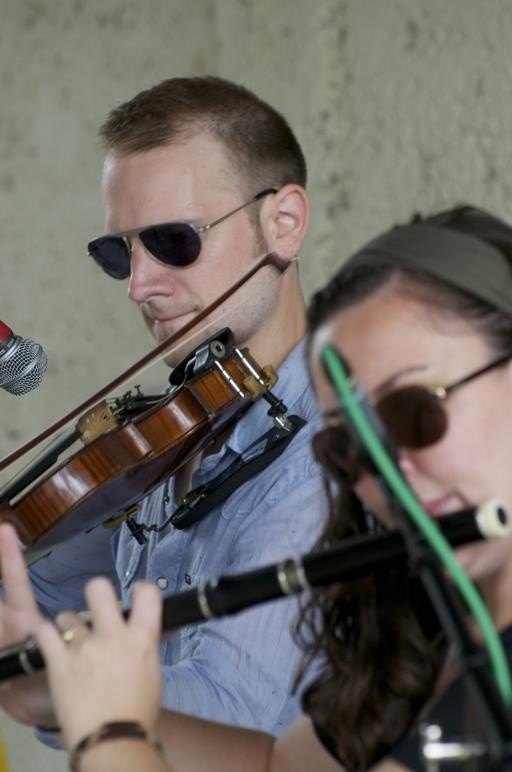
[87,188,277,279]
[313,353,511,487]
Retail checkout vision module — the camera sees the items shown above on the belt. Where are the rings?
[62,618,82,644]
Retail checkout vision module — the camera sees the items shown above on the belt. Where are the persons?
[0,75,342,752]
[1,206,511,772]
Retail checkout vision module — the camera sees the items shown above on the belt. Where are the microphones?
[0,320,48,397]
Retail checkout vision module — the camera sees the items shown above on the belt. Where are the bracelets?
[68,720,164,770]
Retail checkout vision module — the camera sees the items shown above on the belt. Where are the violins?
[0,327,307,554]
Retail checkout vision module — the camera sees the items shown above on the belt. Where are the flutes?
[0,496,511,682]
